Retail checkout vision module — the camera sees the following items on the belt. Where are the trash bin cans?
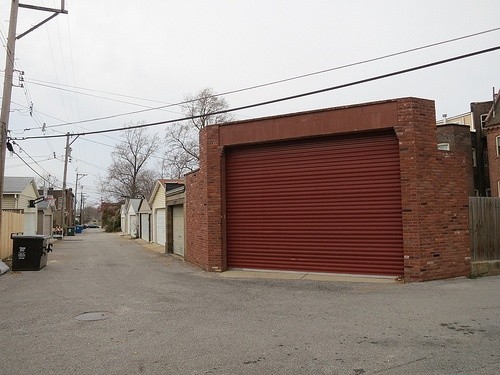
[66,226,75,236]
[11,234,48,271]
[75,226,81,233]
